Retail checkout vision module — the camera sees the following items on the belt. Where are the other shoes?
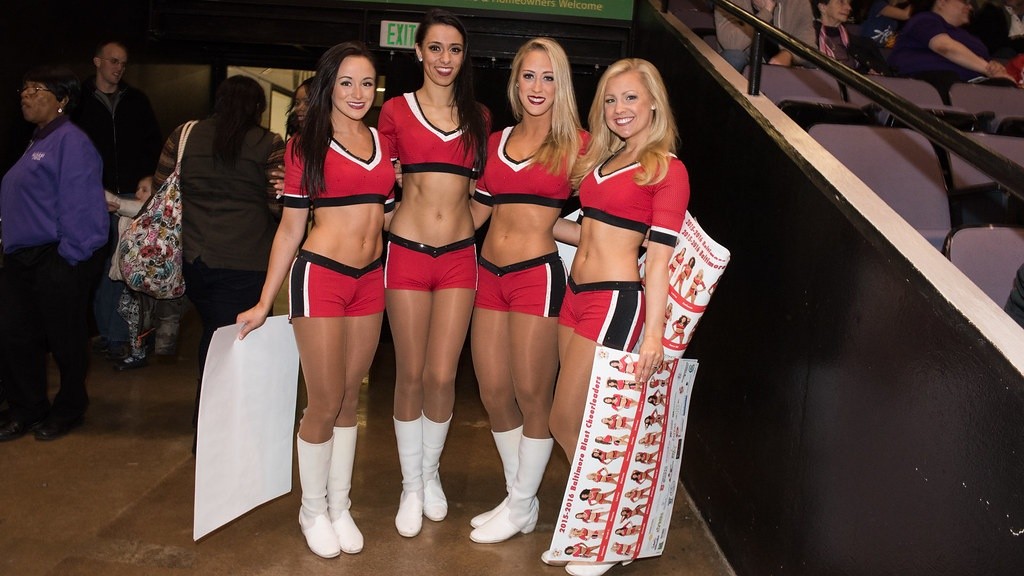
[540,551,567,566]
[156,355,176,364]
[112,354,148,371]
[565,561,633,576]
[104,343,130,359]
[93,339,109,352]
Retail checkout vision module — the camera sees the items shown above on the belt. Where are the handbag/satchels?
[118,119,200,299]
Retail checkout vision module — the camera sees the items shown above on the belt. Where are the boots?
[422,409,453,521]
[326,422,364,554]
[469,432,554,544]
[297,432,340,558]
[393,413,423,538]
[469,424,539,529]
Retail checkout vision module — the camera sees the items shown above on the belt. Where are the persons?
[269,15,492,538]
[394,38,651,542]
[285,76,318,140]
[66,41,154,377]
[0,70,111,444]
[236,9,403,558]
[150,75,287,457]
[549,59,690,576]
[714,0,1024,108]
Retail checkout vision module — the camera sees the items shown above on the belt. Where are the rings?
[651,364,659,369]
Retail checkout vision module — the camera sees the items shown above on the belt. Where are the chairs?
[670,0,1024,309]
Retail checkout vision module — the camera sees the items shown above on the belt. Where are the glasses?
[16,85,50,96]
[97,55,127,69]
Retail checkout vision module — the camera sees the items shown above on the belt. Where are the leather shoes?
[0,416,38,440]
[35,409,85,439]
